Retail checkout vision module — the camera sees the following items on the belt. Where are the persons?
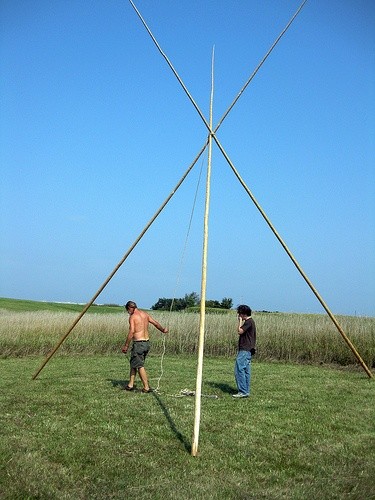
[232,305,256,397]
[122,301,169,393]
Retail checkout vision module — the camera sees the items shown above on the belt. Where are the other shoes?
[232,393,247,398]
[142,388,153,393]
[125,386,133,390]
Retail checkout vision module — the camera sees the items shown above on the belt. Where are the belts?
[134,340,149,342]
